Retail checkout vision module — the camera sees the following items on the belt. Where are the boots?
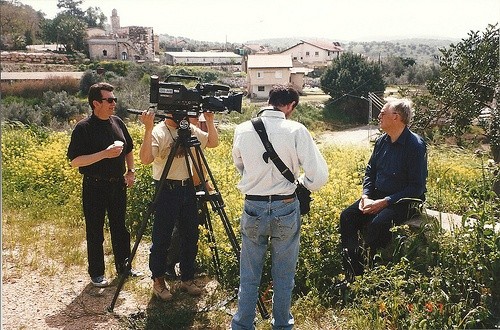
[180,281,201,295]
[152,276,174,302]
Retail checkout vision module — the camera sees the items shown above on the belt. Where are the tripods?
[107,109,270,321]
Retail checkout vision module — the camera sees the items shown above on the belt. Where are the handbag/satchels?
[295,183,314,214]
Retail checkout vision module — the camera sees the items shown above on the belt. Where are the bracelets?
[205,180,208,183]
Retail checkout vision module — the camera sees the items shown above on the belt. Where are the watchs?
[128,168,136,173]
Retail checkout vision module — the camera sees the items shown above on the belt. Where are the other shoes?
[90,276,108,287]
[119,270,144,280]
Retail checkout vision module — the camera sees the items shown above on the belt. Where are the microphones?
[201,83,230,92]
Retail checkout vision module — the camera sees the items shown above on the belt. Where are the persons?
[139,109,220,302]
[67,83,144,287]
[335,97,428,293]
[231,84,329,330]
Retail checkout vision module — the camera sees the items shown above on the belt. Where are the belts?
[245,194,296,201]
[165,178,193,187]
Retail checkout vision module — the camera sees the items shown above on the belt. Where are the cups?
[364,199,374,211]
[114,141,123,146]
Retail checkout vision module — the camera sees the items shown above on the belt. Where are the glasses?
[100,97,117,103]
[381,113,400,117]
